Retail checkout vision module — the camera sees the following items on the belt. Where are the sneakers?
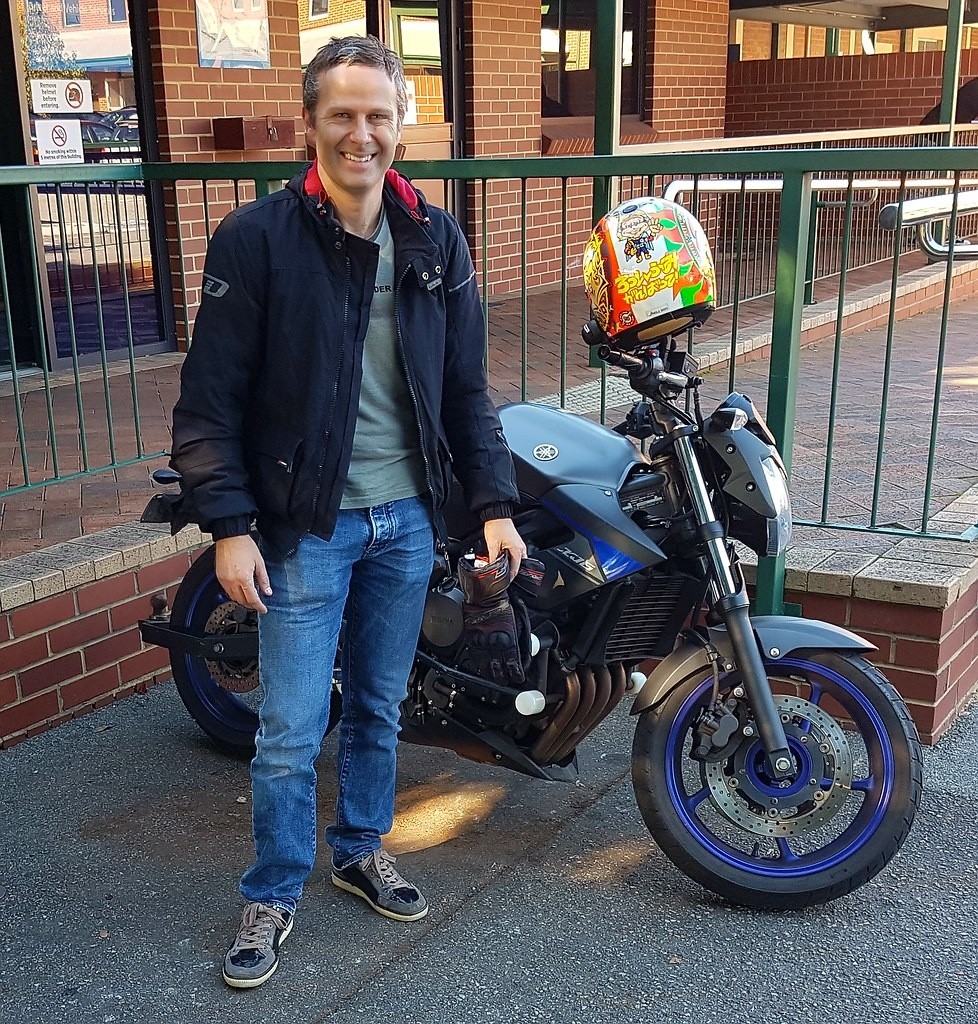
[331,851,430,922]
[221,901,295,989]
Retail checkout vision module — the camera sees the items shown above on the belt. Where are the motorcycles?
[133,332,922,910]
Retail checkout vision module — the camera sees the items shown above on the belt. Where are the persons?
[170,37,528,992]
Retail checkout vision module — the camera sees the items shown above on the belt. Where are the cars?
[30,105,140,147]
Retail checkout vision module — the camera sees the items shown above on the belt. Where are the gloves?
[457,553,544,683]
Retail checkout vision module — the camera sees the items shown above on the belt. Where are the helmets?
[583,197,717,341]
